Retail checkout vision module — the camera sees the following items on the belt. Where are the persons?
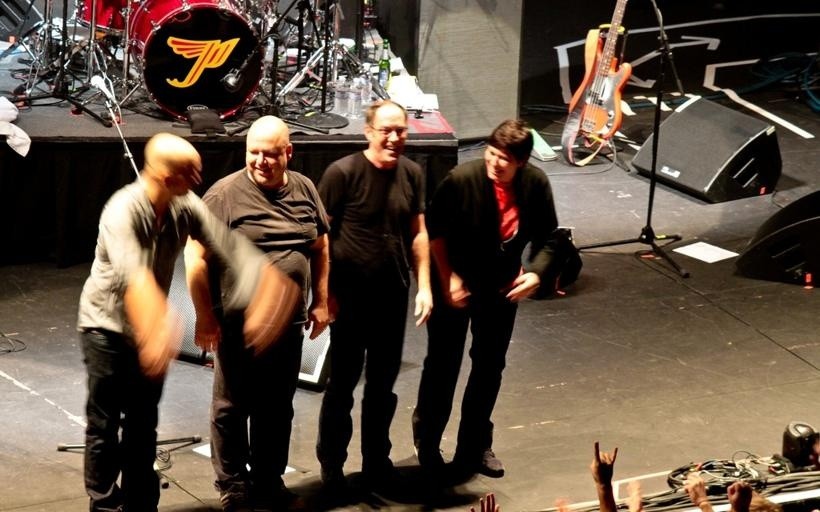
[75,130,301,511]
[317,98,436,510]
[427,117,566,511]
[182,115,329,512]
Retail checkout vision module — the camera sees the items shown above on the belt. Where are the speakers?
[734,189,820,289]
[206,292,334,389]
[168,245,204,359]
[630,95,782,204]
[414,1,524,142]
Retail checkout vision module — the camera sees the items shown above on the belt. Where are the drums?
[76,2,139,36]
[130,1,265,122]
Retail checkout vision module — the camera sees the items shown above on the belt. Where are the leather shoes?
[414,444,505,479]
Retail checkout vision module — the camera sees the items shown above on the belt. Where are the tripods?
[575,0,690,278]
[57,99,202,488]
[0,1,390,131]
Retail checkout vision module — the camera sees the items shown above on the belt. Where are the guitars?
[568,0,633,140]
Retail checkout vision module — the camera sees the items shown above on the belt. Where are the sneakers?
[218,482,306,511]
